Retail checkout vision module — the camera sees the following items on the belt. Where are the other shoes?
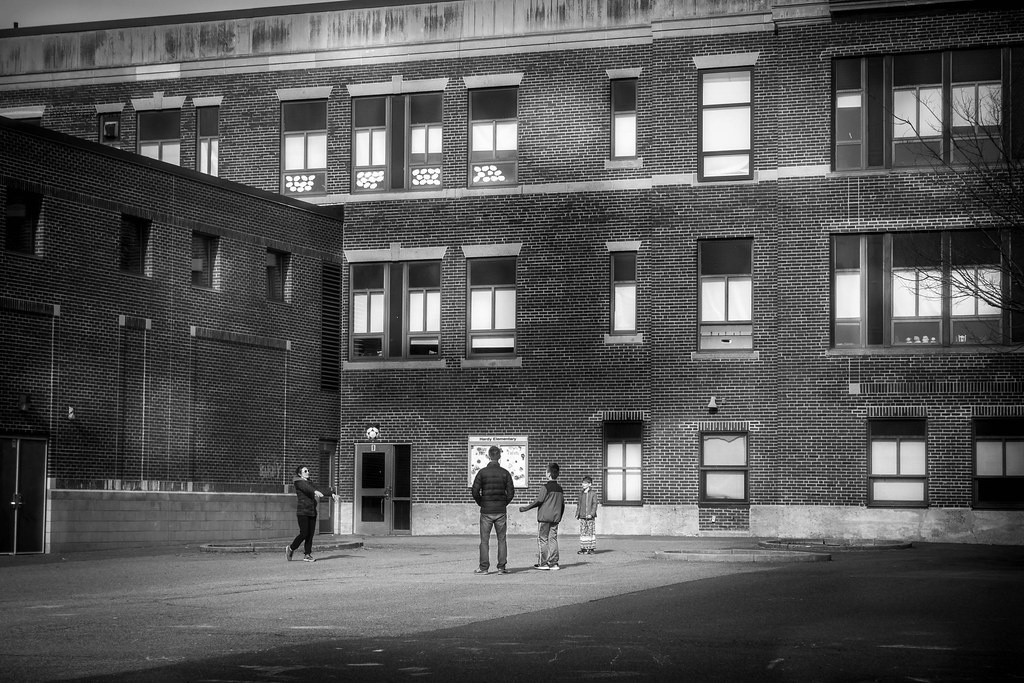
[550,564,559,570]
[578,549,587,555]
[588,549,595,554]
[533,564,550,570]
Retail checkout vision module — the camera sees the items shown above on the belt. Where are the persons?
[575,476,598,555]
[286,465,340,562]
[472,446,514,575]
[519,463,564,570]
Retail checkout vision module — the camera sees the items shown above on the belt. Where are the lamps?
[68,406,76,420]
[19,395,29,411]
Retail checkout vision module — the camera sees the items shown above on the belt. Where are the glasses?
[302,470,309,473]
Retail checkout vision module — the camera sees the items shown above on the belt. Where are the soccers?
[366,427,380,440]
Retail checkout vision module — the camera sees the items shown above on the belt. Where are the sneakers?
[286,545,293,561]
[498,569,512,575]
[474,567,488,575]
[304,555,316,562]
